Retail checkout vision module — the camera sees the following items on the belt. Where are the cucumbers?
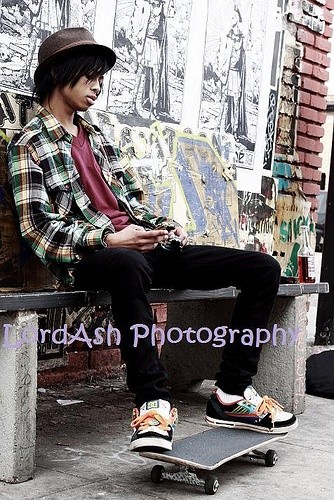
[140,427,288,495]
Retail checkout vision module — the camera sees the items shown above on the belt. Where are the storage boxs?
[1,282,329,483]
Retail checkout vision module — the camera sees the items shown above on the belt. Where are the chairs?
[205,386,298,434]
[129,399,177,452]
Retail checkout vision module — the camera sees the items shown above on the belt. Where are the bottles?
[34,28,116,83]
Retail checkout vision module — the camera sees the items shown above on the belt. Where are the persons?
[215,11,247,140]
[6,27,299,451]
[131,0,171,119]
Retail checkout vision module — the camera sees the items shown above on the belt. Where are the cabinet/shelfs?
[296,226,316,284]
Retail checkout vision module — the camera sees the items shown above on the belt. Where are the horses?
[151,224,174,231]
